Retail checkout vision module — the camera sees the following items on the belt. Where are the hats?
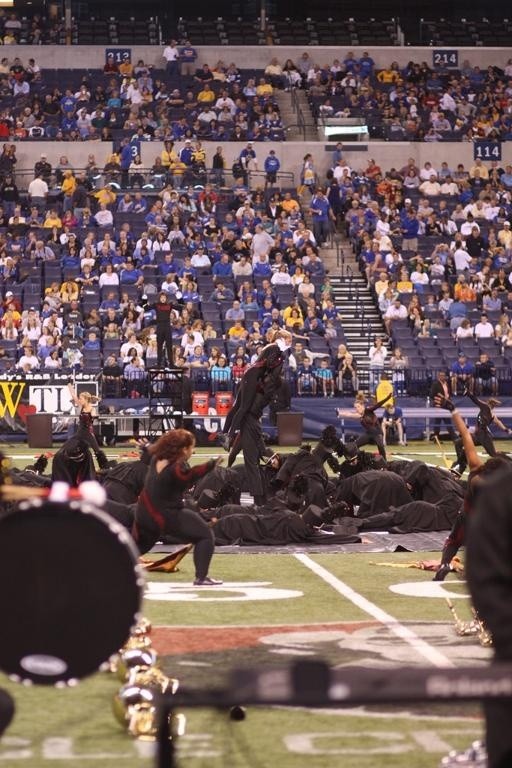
[335,439,358,460]
[289,475,310,509]
[302,500,351,525]
[41,153,48,158]
[504,221,510,225]
[405,198,411,203]
[312,426,340,460]
[197,245,204,249]
[367,159,375,163]
[65,438,85,459]
[198,481,234,508]
[6,290,13,297]
[185,139,191,143]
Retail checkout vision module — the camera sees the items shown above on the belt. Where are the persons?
[2,330,512,584]
[1,137,512,397]
[460,450,510,768]
[2,11,510,143]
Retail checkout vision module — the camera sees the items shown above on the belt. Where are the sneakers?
[193,577,223,585]
[333,516,364,534]
[433,563,450,581]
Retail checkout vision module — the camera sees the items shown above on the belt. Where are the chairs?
[0,0,512,443]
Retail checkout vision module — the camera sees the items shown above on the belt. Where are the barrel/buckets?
[215,391,233,416]
[191,391,209,416]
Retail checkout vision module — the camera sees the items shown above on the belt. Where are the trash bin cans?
[24,413,58,448]
[276,412,304,446]
